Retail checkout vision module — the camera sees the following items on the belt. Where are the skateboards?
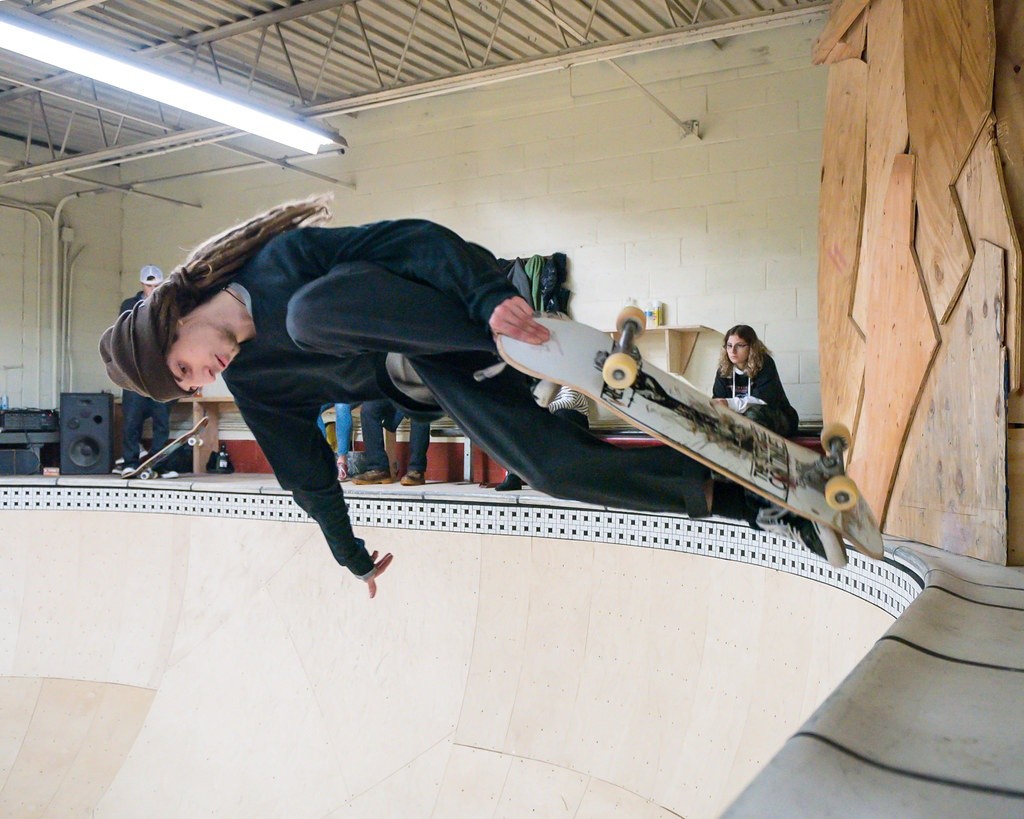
[494,305,885,560]
[120,416,211,480]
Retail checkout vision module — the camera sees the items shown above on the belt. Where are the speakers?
[59,391,114,474]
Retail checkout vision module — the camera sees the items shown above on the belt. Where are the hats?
[99,298,199,404]
[140,266,164,285]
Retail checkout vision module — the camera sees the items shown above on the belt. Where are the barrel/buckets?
[347,451,367,477]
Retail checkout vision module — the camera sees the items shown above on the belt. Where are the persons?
[711,324,798,435]
[98,198,849,599]
[117,266,179,478]
[495,383,590,491]
[317,400,431,485]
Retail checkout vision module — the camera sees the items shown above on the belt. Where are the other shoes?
[352,470,393,485]
[111,459,122,474]
[121,465,136,477]
[115,443,149,465]
[495,473,521,491]
[401,468,425,485]
[153,465,178,478]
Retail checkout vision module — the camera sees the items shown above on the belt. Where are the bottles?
[219,445,227,468]
[624,297,632,307]
[0,394,9,410]
[645,301,662,329]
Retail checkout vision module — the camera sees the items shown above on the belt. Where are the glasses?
[726,344,750,351]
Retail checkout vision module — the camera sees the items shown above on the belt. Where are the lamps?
[0,11,349,154]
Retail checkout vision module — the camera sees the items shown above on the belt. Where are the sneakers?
[747,490,849,566]
[527,373,557,408]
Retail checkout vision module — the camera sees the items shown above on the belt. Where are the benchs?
[117,397,822,488]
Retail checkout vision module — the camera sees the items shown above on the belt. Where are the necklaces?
[222,288,246,305]
